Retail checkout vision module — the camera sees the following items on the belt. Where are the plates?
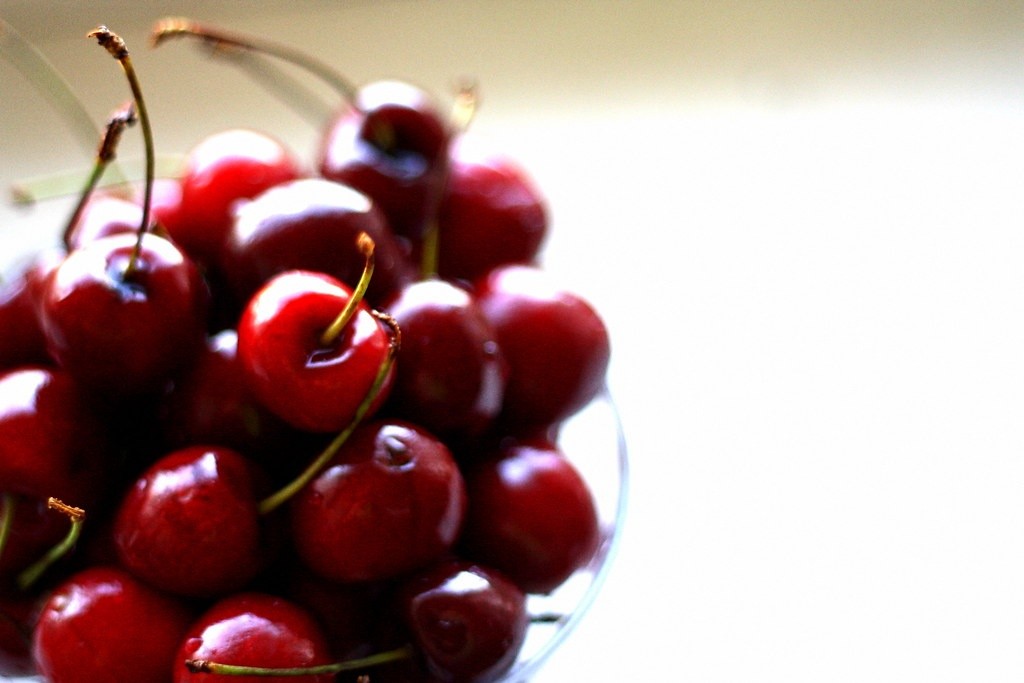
[0,180,630,683]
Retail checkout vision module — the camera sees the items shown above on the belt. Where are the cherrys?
[0,19,610,683]
